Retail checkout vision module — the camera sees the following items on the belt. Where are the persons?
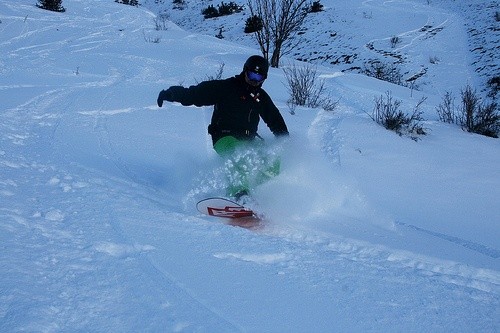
[156,55,290,205]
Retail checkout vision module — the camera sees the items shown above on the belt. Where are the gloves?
[157,89,173,107]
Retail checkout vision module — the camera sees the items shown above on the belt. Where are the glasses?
[244,65,266,81]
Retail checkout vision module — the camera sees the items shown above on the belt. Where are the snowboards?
[196,197,253,219]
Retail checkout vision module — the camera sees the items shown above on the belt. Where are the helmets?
[243,55,269,77]
